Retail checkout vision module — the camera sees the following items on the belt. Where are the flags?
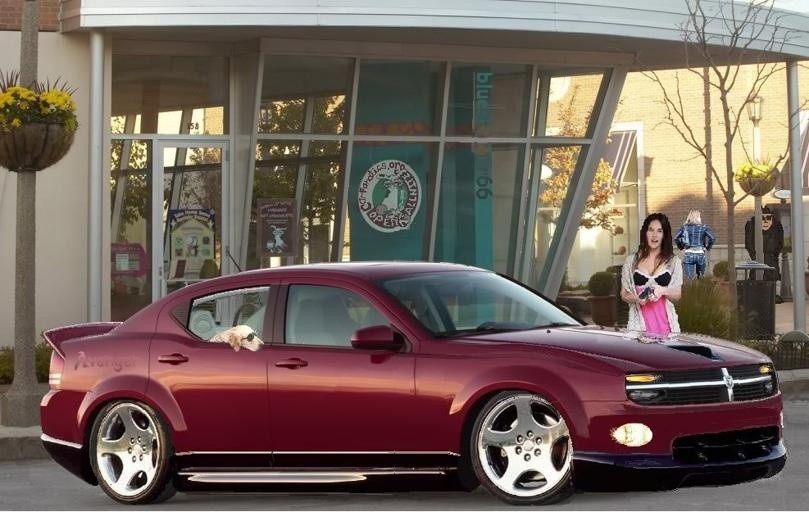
[740,177,777,198]
[0,124,73,172]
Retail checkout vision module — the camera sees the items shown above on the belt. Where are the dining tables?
[586,272,618,324]
[804,256,809,292]
[712,260,737,305]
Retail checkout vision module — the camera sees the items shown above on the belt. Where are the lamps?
[325,293,359,332]
[188,309,217,340]
[288,299,335,345]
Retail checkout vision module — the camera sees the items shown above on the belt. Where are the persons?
[676,209,715,281]
[620,213,684,335]
[745,205,784,302]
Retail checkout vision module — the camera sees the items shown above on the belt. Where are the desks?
[745,95,766,279]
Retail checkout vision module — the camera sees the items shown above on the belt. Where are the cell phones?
[639,287,650,298]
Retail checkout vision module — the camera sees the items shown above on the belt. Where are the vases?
[734,153,774,183]
[0,69,80,145]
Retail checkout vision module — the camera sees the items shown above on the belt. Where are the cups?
[243,331,257,341]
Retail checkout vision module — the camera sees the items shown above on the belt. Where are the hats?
[39,260,788,506]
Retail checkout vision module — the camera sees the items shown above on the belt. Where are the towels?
[736,261,775,340]
